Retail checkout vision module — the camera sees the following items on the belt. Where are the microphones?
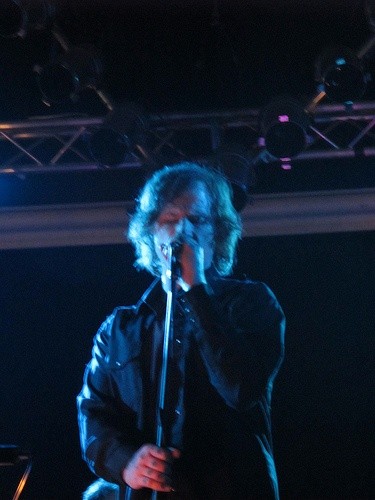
[168,242,184,254]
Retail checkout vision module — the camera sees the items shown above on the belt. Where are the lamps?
[258,96,312,165]
[213,141,254,213]
[312,46,366,103]
[85,105,146,164]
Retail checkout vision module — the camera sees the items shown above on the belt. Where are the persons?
[75,161,285,500]
